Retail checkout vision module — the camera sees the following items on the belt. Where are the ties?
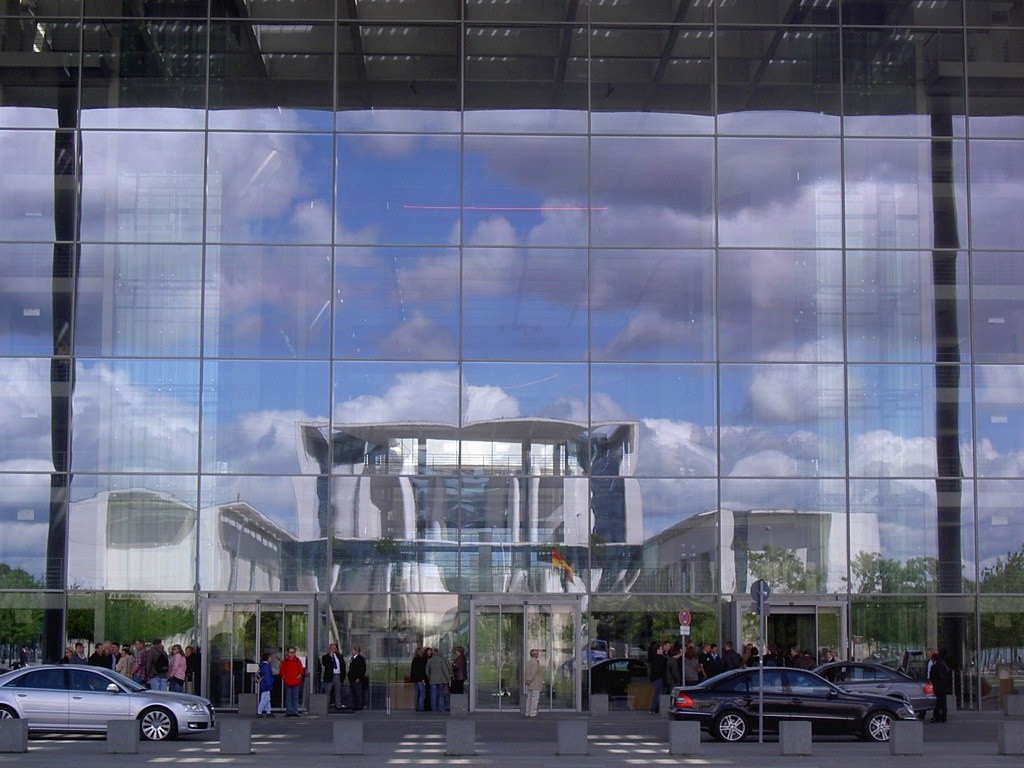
[333,654,337,669]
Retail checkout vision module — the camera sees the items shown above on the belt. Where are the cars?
[0,663,216,742]
[561,639,651,703]
[741,659,937,721]
[667,665,918,743]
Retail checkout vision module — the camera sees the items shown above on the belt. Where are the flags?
[553,549,576,587]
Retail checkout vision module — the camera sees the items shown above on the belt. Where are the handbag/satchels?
[300,676,305,686]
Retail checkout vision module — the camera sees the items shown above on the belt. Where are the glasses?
[289,651,295,653]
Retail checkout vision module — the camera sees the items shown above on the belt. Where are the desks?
[390,683,448,711]
[627,684,655,710]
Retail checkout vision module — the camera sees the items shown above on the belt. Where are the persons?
[279,647,305,718]
[992,652,1005,677]
[650,640,840,717]
[410,645,451,712]
[446,646,467,712]
[62,638,196,696]
[347,646,366,711]
[496,652,512,698]
[926,654,951,723]
[917,648,937,721]
[256,653,276,717]
[322,644,345,710]
[21,644,30,668]
[525,649,542,717]
[607,647,616,659]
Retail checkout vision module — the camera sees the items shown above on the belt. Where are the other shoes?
[266,714,276,717]
[284,712,300,718]
[256,713,264,718]
